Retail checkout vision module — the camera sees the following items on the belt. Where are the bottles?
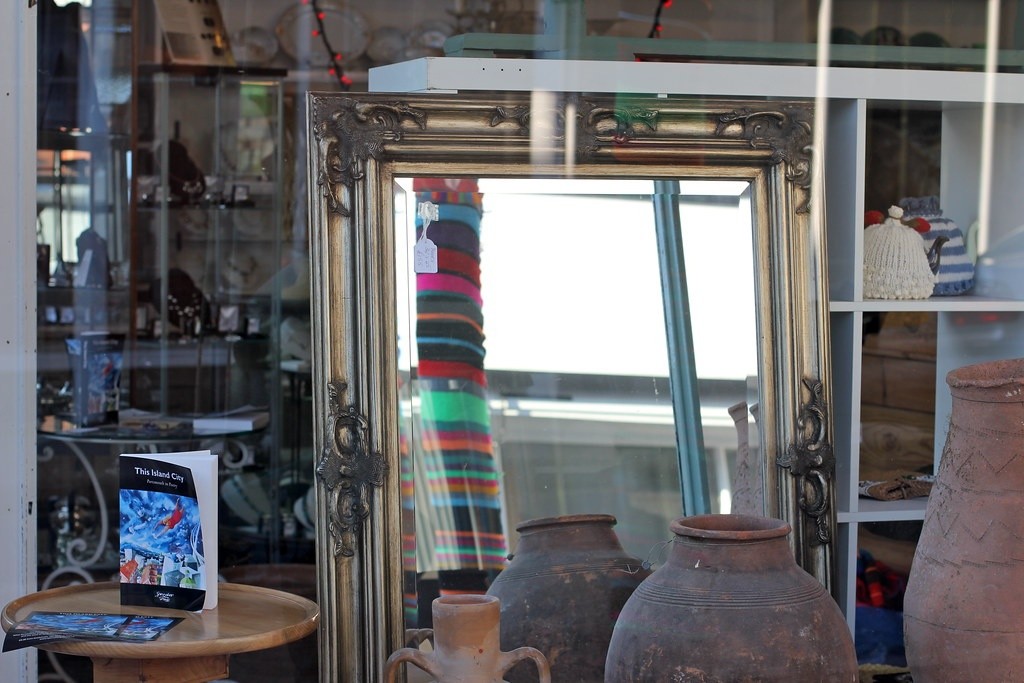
[180,570,196,589]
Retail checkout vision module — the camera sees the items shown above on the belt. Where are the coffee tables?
[0,579,321,683]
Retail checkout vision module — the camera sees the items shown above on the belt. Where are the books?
[65,331,125,433]
[193,403,273,432]
[119,448,218,614]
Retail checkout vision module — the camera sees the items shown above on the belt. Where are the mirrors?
[307,94,838,683]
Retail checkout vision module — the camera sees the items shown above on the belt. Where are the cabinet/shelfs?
[367,55,1024,683]
[37,63,295,580]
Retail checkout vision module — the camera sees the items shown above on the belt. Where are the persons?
[151,498,185,538]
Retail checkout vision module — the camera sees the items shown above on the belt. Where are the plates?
[406,20,455,49]
[230,27,278,65]
[367,26,404,63]
[274,0,373,66]
